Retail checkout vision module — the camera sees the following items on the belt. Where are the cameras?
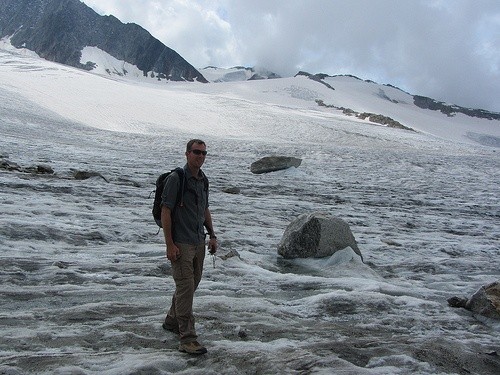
[210,247,216,254]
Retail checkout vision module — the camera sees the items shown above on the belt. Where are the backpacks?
[149,168,208,229]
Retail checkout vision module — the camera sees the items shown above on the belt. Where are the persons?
[160,139,217,356]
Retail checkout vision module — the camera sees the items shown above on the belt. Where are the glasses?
[188,149,208,155]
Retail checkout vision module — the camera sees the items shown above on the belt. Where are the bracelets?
[209,235,216,239]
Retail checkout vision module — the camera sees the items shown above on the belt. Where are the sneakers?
[162,322,183,335]
[179,340,208,354]
